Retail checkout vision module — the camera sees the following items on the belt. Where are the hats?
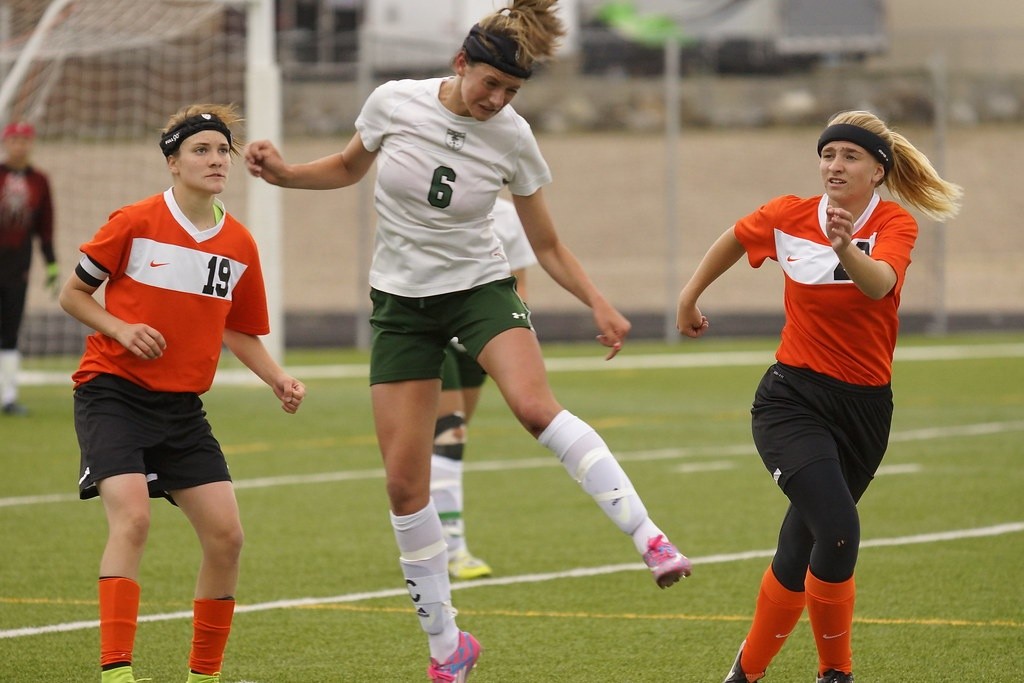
[3,119,36,142]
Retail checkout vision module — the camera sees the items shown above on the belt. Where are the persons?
[60,104,303,682]
[428,197,538,577]
[246,0,693,683]
[0,122,60,417]
[675,110,957,682]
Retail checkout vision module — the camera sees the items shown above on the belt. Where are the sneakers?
[816,668,854,683]
[723,639,766,683]
[641,534,691,588]
[428,631,481,683]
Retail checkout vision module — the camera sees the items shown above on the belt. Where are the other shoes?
[447,547,492,579]
[0,385,16,407]
[101,666,152,683]
[187,668,221,683]
